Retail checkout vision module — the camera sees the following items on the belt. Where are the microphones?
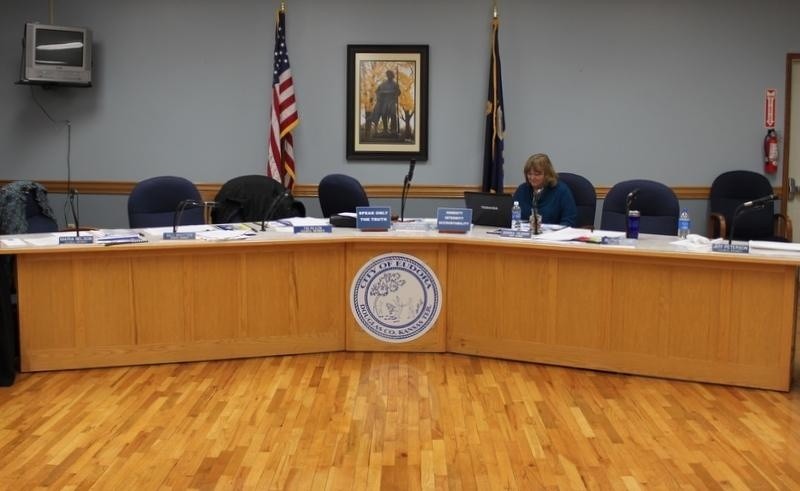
[162,200,220,240]
[624,187,643,237]
[393,156,427,231]
[59,187,93,243]
[261,189,291,232]
[712,193,779,254]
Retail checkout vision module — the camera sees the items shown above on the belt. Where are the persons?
[375,70,401,133]
[513,153,577,228]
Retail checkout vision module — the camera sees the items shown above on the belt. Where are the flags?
[482,1,506,192]
[268,2,299,197]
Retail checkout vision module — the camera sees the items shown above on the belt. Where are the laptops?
[464,191,513,229]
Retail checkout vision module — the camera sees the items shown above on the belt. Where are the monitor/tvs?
[20,22,92,85]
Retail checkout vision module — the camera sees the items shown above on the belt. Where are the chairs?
[557,172,597,230]
[127,176,205,229]
[210,175,306,224]
[0,181,58,234]
[318,174,371,218]
[600,180,680,236]
[710,170,774,241]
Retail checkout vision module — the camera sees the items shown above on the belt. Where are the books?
[282,211,332,231]
[523,221,626,244]
[193,222,272,243]
[92,228,148,247]
[671,233,800,259]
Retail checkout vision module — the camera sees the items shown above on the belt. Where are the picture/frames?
[346,45,430,161]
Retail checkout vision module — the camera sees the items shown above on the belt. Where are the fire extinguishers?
[764,129,778,174]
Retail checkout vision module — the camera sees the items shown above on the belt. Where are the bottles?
[677,207,692,241]
[511,199,523,232]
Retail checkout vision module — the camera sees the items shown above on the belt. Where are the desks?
[0,217,800,392]
